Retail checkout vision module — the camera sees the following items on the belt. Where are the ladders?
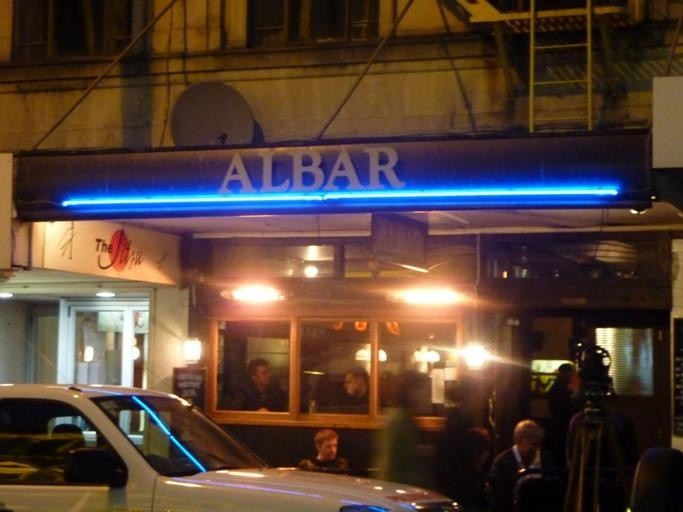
[529,0,593,133]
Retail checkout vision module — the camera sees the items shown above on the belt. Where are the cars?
[0,377,466,512]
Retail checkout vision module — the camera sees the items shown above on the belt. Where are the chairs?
[53,423,86,468]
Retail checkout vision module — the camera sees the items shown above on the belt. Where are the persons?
[543,362,579,449]
[231,358,289,411]
[488,420,550,505]
[323,366,383,414]
[298,427,349,475]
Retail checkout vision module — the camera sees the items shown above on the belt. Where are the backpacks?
[509,445,548,509]
